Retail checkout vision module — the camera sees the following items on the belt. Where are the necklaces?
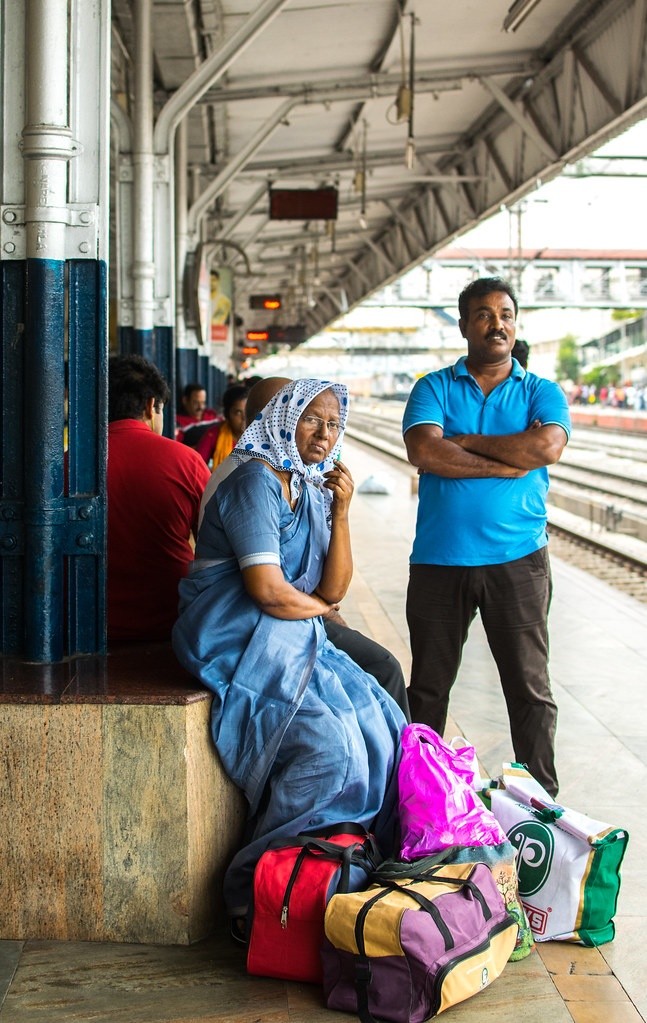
[279,472,295,512]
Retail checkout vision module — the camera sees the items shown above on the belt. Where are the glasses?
[300,415,345,432]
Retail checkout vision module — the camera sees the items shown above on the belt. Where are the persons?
[64,357,295,676]
[171,378,409,944]
[563,381,647,411]
[511,339,530,371]
[402,278,572,802]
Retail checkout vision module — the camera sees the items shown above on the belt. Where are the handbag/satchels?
[323,861,519,1023]
[475,762,629,948]
[374,842,536,962]
[398,723,509,861]
[448,736,490,792]
[242,822,385,982]
